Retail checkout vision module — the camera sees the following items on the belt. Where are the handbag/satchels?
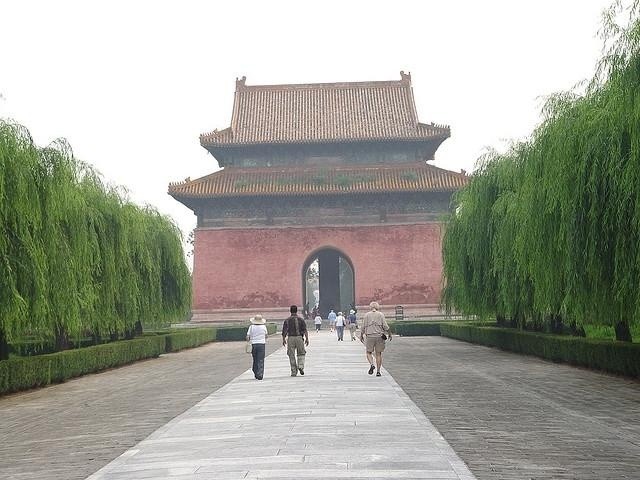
[246,341,252,353]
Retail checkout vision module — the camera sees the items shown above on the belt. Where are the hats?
[249,315,266,325]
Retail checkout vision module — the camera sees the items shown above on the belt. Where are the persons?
[282,305,309,377]
[314,314,322,333]
[327,309,336,333]
[347,309,356,341]
[246,314,268,380]
[334,312,346,341]
[359,302,393,376]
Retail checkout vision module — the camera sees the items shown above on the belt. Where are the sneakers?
[290,367,304,376]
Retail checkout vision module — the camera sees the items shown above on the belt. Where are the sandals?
[368,364,381,377]
